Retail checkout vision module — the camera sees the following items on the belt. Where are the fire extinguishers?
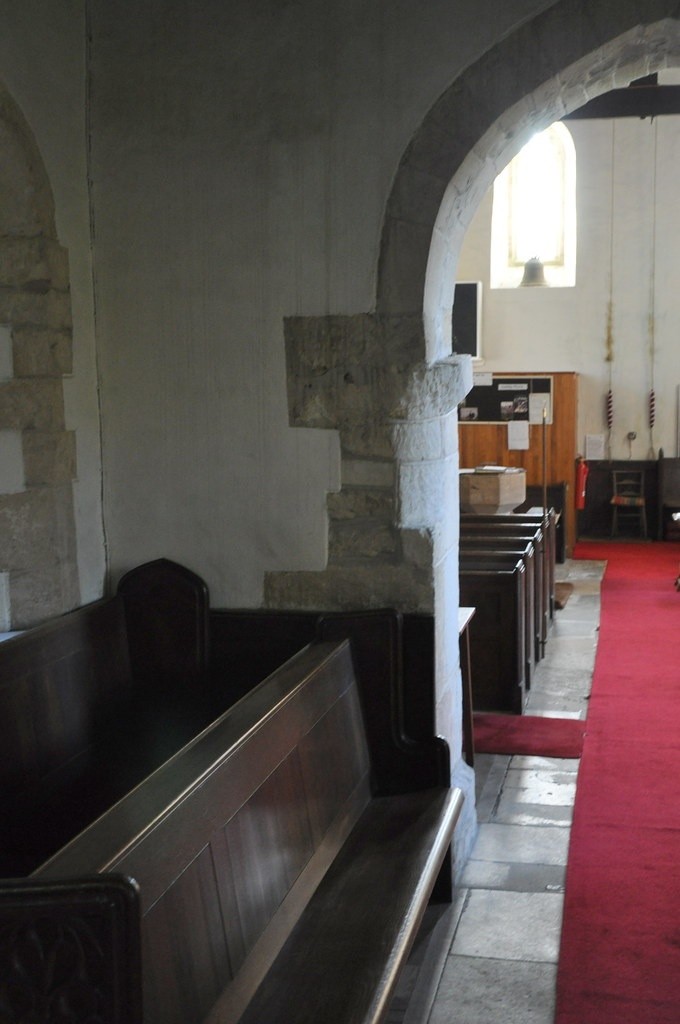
[574,455,590,510]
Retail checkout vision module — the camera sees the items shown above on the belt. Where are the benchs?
[0,557,471,1024]
[657,448,680,539]
[459,482,567,716]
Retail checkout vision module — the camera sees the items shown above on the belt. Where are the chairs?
[609,470,647,539]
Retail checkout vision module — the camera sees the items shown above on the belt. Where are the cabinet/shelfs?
[457,372,579,555]
